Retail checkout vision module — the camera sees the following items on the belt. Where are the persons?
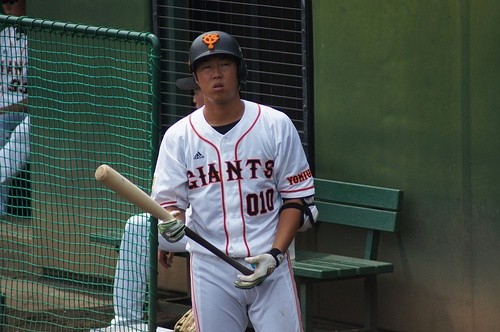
[0,0,30,221]
[89,74,206,332]
[144,31,319,332]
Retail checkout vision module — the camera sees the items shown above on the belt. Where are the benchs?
[87,177,403,332]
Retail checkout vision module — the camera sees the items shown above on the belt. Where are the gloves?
[157,209,186,244]
[234,248,284,290]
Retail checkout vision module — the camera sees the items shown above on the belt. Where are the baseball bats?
[94,164,254,275]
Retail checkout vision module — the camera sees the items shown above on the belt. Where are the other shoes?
[89,323,123,332]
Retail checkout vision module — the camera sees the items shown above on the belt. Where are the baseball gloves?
[173,307,197,331]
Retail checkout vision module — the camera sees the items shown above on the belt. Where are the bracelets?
[268,248,284,269]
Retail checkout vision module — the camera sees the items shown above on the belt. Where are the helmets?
[187,30,249,91]
